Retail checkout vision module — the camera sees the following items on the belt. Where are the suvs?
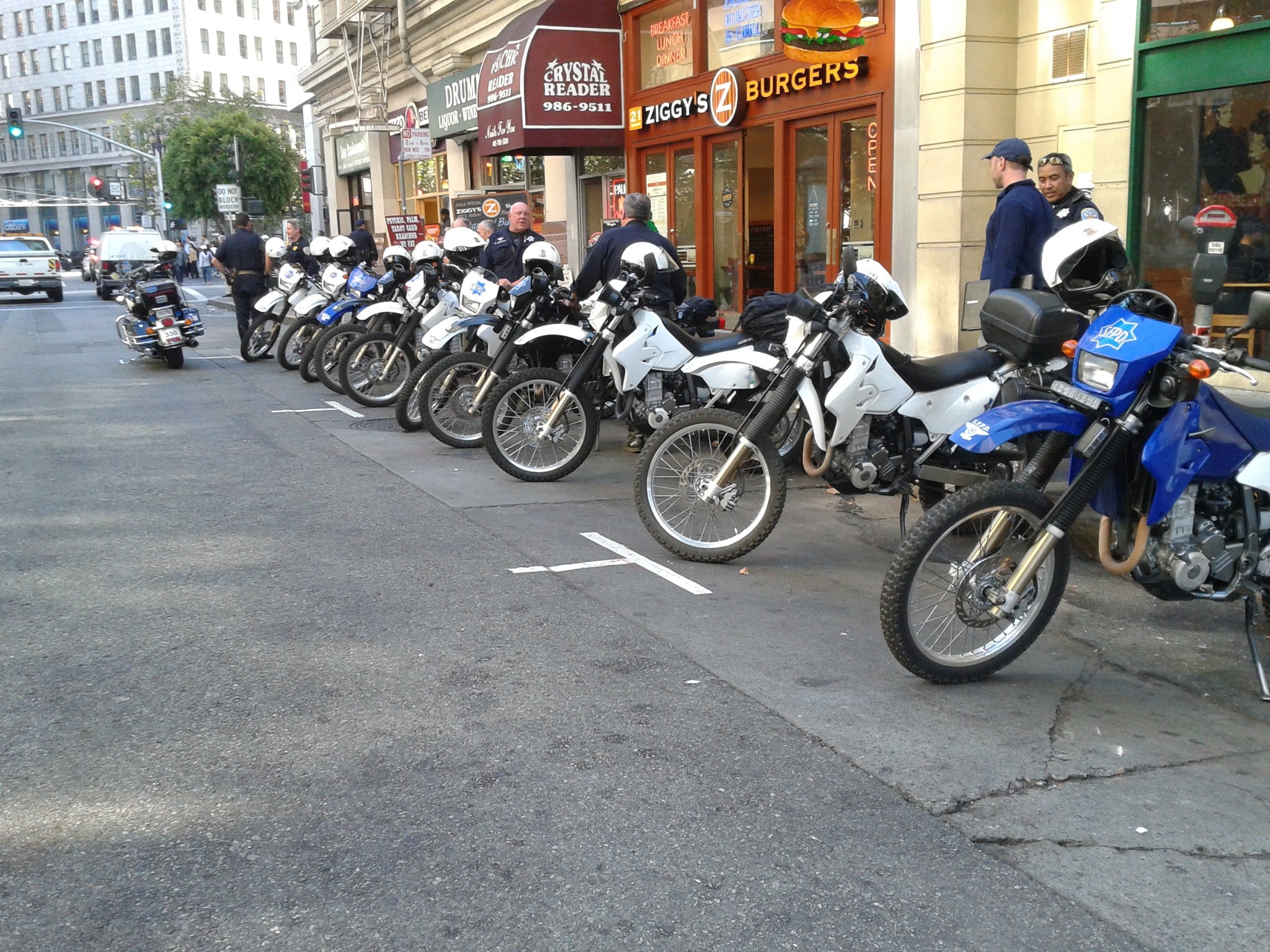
[89,226,172,301]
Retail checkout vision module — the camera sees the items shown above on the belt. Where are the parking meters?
[1192,205,1238,347]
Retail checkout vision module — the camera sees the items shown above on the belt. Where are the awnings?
[478,0,625,156]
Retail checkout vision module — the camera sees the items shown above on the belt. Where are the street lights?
[148,118,168,240]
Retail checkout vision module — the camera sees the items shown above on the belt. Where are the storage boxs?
[979,288,1078,363]
[124,316,154,349]
[137,279,178,309]
[182,303,205,338]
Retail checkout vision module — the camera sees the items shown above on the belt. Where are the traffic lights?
[210,188,218,205]
[88,178,108,198]
[6,107,24,140]
[301,167,315,194]
[163,192,172,209]
[169,220,179,229]
[226,143,237,179]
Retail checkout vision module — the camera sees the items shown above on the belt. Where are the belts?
[237,270,264,277]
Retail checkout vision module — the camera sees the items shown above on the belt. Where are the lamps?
[1210,4,1239,31]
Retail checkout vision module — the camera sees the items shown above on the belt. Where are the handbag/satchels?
[736,291,827,342]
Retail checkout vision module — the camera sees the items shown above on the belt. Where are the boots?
[622,423,646,453]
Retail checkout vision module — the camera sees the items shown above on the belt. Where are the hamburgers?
[780,0,865,64]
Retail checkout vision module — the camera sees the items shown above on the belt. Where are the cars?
[0,236,63,302]
[54,248,97,282]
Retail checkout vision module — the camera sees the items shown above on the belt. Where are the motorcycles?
[879,288,1269,702]
[239,234,1080,565]
[110,241,207,370]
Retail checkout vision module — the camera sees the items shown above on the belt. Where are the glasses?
[1038,157,1071,168]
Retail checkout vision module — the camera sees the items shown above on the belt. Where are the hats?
[980,139,1034,171]
[356,219,365,226]
[200,245,207,250]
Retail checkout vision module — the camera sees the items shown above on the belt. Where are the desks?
[814,271,826,278]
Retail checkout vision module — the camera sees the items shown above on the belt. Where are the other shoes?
[223,294,232,297]
[516,395,530,413]
[205,282,212,285]
[260,354,274,359]
[183,273,199,279]
[178,282,183,286]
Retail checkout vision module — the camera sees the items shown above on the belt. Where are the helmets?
[620,243,668,273]
[1042,218,1132,309]
[157,240,177,263]
[310,237,333,264]
[412,240,444,269]
[444,227,487,267]
[382,245,412,272]
[265,237,288,260]
[522,241,561,280]
[328,235,359,265]
[834,258,909,320]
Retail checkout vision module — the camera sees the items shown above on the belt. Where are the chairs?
[797,259,813,290]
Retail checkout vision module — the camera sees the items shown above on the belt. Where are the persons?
[348,220,378,269]
[172,234,226,286]
[480,202,547,302]
[442,217,471,269]
[477,219,497,242]
[565,192,688,452]
[439,208,451,238]
[285,218,310,252]
[212,212,274,359]
[1038,152,1104,237]
[979,138,1055,349]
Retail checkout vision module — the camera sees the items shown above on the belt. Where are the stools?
[1209,309,1265,359]
